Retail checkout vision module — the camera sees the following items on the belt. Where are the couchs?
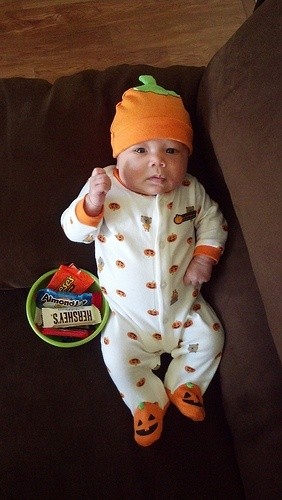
[1,0,282,500]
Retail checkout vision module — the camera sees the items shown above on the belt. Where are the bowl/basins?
[24,268,109,347]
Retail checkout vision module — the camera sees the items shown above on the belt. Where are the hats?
[109,75,192,157]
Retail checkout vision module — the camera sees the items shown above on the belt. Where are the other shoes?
[133,382,206,448]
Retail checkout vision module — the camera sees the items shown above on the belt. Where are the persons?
[60,75,228,446]
